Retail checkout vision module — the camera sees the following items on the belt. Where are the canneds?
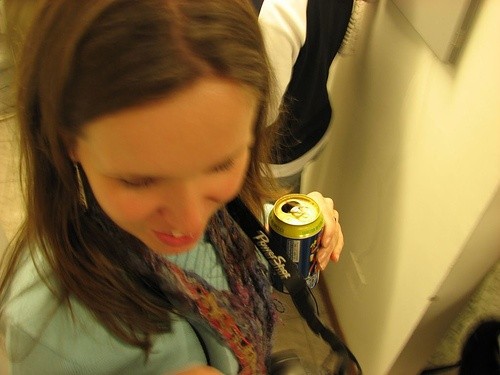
[267,194,325,295]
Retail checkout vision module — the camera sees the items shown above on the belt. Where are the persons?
[1,0,344,375]
[251,0,353,201]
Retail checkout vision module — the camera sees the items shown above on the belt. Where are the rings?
[333,215,337,220]
